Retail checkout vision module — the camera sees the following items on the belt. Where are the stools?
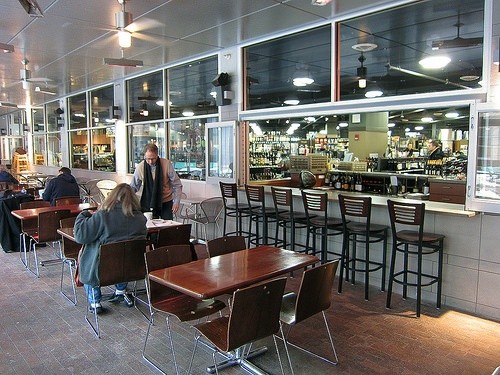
[218,179,446,319]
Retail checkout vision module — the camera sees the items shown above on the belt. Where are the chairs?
[0,173,340,375]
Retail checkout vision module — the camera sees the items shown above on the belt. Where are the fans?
[88,0,173,47]
[342,54,398,86]
[0,59,54,88]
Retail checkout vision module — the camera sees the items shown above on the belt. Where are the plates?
[152,218,165,224]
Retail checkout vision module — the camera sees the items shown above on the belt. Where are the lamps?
[118,31,131,47]
[284,47,453,104]
[0,128,6,136]
[215,85,235,106]
[55,116,64,127]
[23,124,30,132]
[22,80,32,90]
[112,105,122,120]
[143,95,195,118]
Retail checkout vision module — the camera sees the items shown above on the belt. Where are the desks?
[10,202,95,266]
[179,196,208,244]
[149,245,320,375]
[56,219,183,303]
[78,182,96,203]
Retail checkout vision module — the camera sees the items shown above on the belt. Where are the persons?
[74,182,147,313]
[130,144,182,221]
[43,167,80,206]
[279,154,291,171]
[0,164,19,184]
[427,141,444,160]
[404,143,413,156]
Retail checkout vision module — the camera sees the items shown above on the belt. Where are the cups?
[143,212,153,220]
[38,189,44,199]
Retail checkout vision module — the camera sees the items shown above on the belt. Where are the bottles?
[324,173,363,192]
[425,165,441,175]
[249,131,348,180]
[21,187,27,194]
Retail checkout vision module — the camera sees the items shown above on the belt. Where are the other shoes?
[88,305,103,314]
[108,292,124,302]
